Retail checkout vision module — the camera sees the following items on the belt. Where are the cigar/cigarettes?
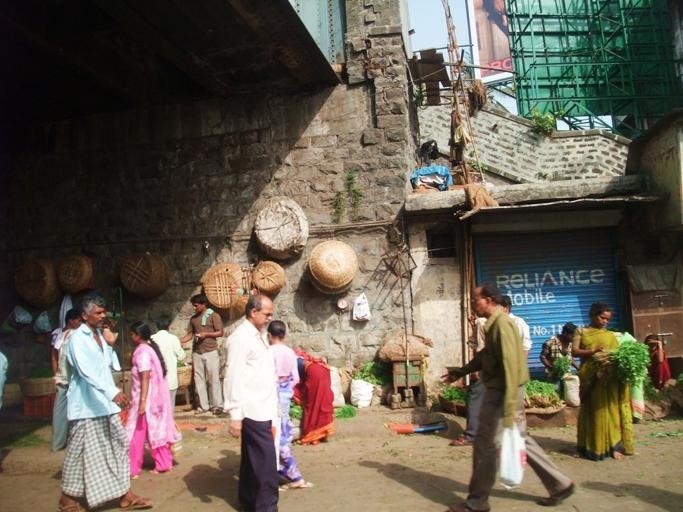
[196,336,199,342]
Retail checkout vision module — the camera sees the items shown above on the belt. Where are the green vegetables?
[595,341,650,386]
[524,355,572,408]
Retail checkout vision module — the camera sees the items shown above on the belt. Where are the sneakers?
[449,434,473,446]
[194,405,205,414]
[212,407,222,414]
[448,502,490,512]
[539,482,574,506]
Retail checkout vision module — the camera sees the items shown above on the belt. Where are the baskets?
[17,377,55,397]
[23,396,53,417]
[177,364,191,388]
[13,257,59,307]
[3,383,23,407]
[202,194,358,320]
[118,249,167,300]
[57,253,94,292]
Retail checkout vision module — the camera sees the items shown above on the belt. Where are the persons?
[573,298,635,460]
[441,283,577,512]
[290,346,336,446]
[449,313,491,448]
[483,0,509,40]
[219,296,284,511]
[60,293,155,512]
[149,313,187,414]
[540,322,581,392]
[177,294,225,416]
[49,307,85,452]
[121,319,182,480]
[498,294,534,361]
[609,326,645,424]
[265,320,307,490]
[644,335,672,392]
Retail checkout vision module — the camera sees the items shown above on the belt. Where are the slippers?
[119,496,152,509]
[58,500,86,512]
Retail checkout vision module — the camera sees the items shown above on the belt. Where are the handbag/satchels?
[169,423,184,457]
